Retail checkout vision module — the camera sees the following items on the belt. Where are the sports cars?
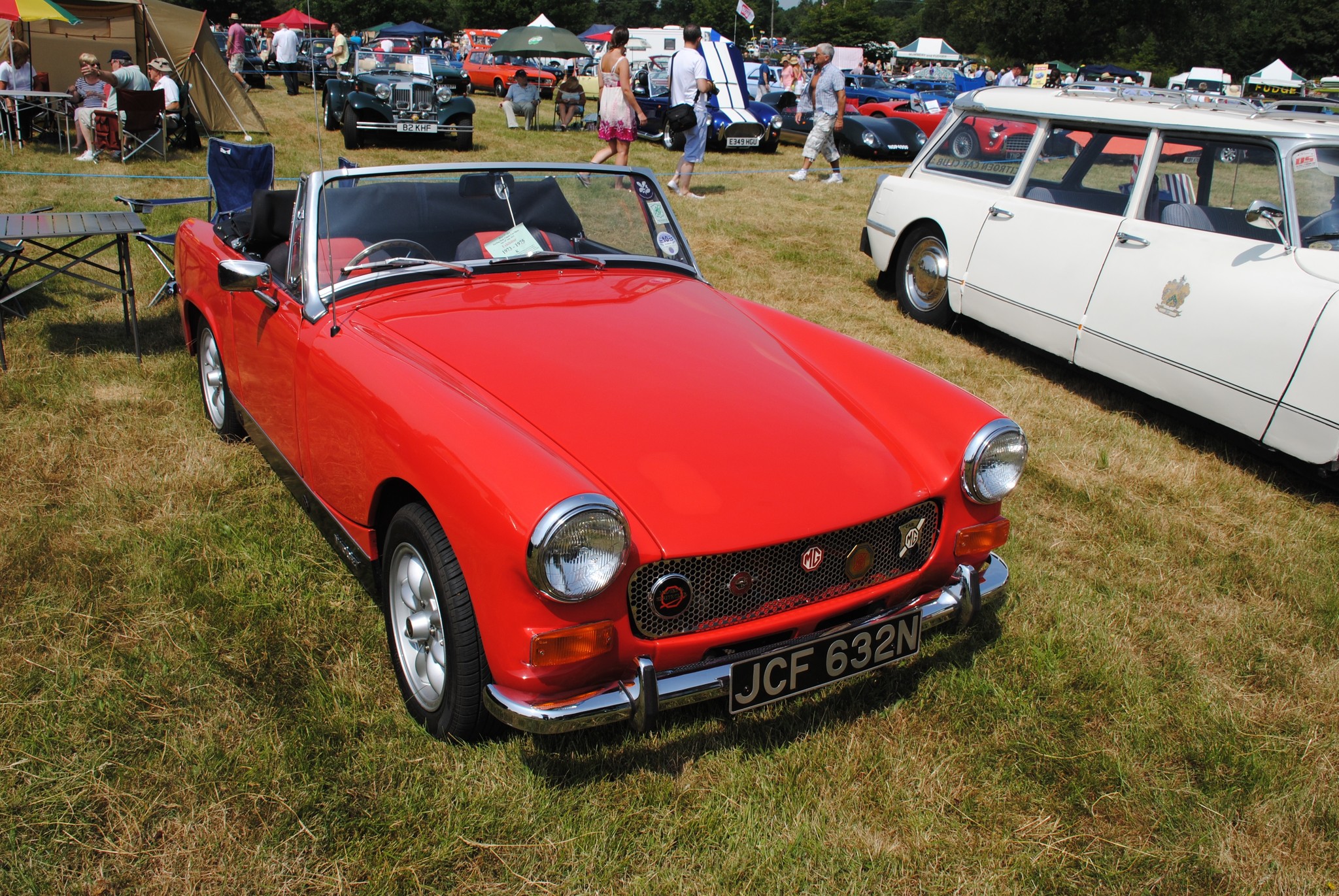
[172,159,1027,748]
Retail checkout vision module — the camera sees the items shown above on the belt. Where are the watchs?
[95,69,101,78]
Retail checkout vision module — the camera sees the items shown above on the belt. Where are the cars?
[859,84,1339,470]
[211,30,1339,168]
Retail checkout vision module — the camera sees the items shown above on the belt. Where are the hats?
[1099,73,1114,81]
[1066,73,1071,76]
[936,62,941,67]
[779,56,791,64]
[915,62,920,66]
[1120,76,1136,85]
[789,57,798,65]
[146,57,173,72]
[956,63,962,67]
[106,50,132,63]
[1014,61,1026,69]
[515,69,529,77]
[1224,84,1241,98]
[228,13,241,20]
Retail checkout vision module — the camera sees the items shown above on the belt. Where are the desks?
[0,90,73,154]
[0,210,147,367]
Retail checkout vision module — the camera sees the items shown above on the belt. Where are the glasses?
[88,62,100,66]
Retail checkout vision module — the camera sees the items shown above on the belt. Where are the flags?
[736,0,754,23]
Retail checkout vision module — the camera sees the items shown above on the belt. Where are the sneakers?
[788,168,807,181]
[243,83,251,93]
[74,150,95,162]
[560,124,567,132]
[679,191,706,199]
[820,173,843,184]
[114,145,132,158]
[666,180,679,194]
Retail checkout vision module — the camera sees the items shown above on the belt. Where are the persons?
[113,58,181,158]
[213,13,363,96]
[755,38,1308,182]
[498,69,541,131]
[444,38,478,63]
[667,25,715,198]
[72,50,152,161]
[555,76,587,131]
[69,52,107,149]
[0,39,43,143]
[577,25,648,194]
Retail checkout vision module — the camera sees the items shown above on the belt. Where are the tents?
[361,21,445,67]
[261,7,330,38]
[0,0,268,134]
[526,14,615,42]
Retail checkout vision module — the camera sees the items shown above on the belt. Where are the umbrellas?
[0,0,85,149]
[584,27,653,64]
[489,25,595,132]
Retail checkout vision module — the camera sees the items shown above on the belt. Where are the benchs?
[1023,186,1130,216]
[1162,203,1308,248]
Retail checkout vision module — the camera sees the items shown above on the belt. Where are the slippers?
[577,173,590,188]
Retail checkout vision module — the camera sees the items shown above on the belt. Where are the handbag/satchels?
[666,103,697,132]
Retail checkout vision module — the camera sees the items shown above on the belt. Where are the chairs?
[454,229,575,262]
[30,72,55,142]
[153,80,190,151]
[335,156,362,189]
[395,62,414,72]
[263,236,395,295]
[358,60,376,73]
[506,95,537,130]
[89,87,167,166]
[111,137,276,307]
[553,99,585,128]
[0,205,56,319]
